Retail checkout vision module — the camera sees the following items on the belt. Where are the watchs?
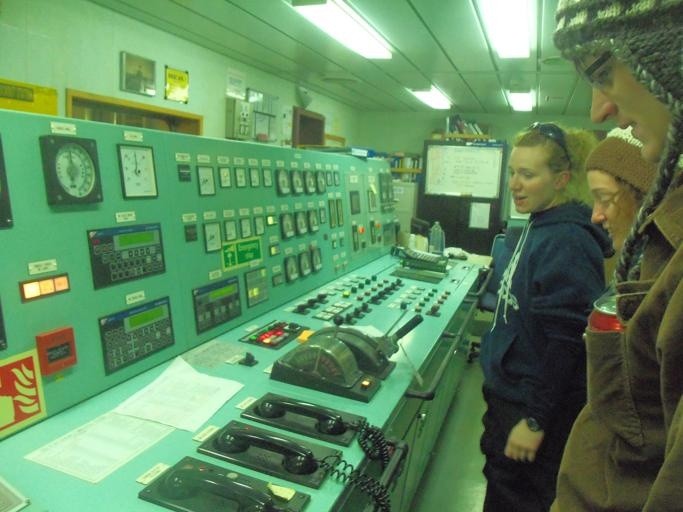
[528,416,540,432]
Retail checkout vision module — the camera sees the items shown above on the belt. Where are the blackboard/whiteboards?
[423,139,507,205]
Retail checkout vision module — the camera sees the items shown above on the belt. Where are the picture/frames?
[118,50,158,97]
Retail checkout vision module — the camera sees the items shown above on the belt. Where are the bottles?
[407,234,415,249]
[428,221,441,252]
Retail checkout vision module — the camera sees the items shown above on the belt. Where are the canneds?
[587,295,623,332]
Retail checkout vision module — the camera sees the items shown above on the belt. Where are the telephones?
[218,426,315,476]
[256,397,347,435]
[163,468,274,512]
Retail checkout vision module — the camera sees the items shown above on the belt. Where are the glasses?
[570,50,615,87]
[530,121,573,172]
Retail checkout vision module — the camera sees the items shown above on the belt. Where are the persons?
[582,126,651,253]
[478,122,612,511]
[543,0,683,512]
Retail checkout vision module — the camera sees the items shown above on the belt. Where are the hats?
[585,123,659,196]
[555,0,683,293]
